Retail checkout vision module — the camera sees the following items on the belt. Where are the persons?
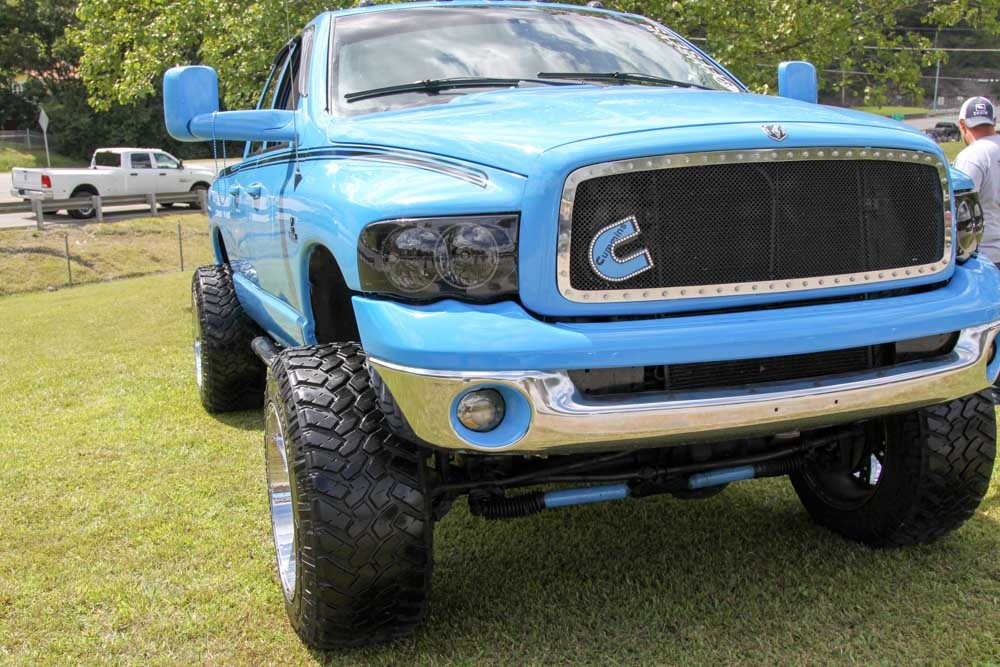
[953,96,999,272]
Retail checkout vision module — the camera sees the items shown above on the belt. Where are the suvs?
[162,1,999,651]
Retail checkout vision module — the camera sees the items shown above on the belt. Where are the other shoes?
[992,385,1000,404]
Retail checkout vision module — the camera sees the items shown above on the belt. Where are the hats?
[959,97,996,128]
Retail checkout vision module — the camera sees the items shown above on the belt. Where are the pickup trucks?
[920,121,961,142]
[9,146,216,220]
